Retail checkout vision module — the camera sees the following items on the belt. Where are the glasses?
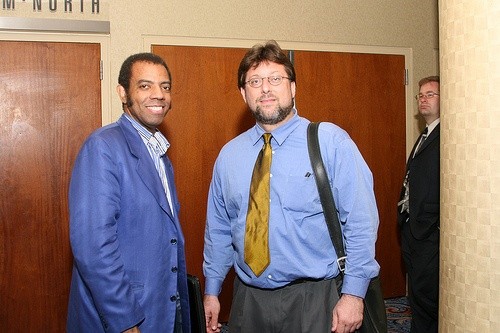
[415,91,439,101]
[242,74,293,88]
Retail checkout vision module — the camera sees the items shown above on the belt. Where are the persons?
[67,52,192,333]
[395,76,441,333]
[207,41,386,333]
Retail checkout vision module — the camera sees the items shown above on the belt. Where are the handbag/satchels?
[338,266,387,333]
[187,273,207,333]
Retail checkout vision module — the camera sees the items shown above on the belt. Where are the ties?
[417,126,429,156]
[243,131,274,277]
